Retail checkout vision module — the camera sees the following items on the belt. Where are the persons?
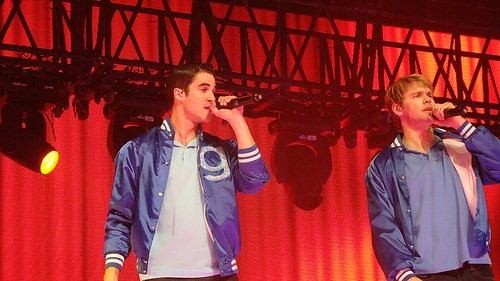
[103,64,270,281]
[364,74,500,281]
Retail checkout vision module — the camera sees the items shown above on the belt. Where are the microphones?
[432,107,468,119]
[215,94,262,111]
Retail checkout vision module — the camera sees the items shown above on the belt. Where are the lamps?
[0,81,332,211]
